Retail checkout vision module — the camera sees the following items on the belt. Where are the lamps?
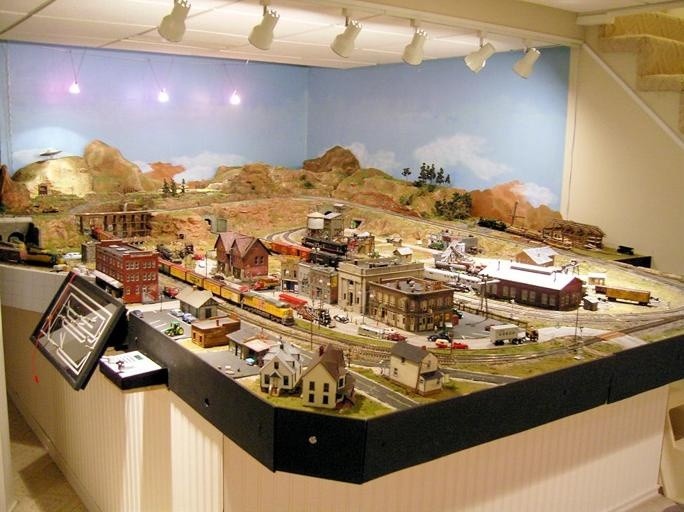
[327,8,363,59]
[467,29,494,74]
[516,34,541,81]
[400,18,429,68]
[157,2,194,44]
[249,2,280,51]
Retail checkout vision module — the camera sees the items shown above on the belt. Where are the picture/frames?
[29,270,125,392]
